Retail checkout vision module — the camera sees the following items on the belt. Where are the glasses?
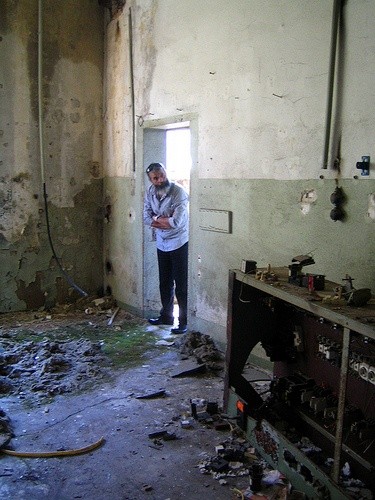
[145,163,161,173]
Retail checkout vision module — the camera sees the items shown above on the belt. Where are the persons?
[143,162,189,334]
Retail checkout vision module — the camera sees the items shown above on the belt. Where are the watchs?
[155,214,162,221]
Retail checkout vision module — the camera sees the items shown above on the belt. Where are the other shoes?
[148,318,173,325]
[171,326,186,334]
